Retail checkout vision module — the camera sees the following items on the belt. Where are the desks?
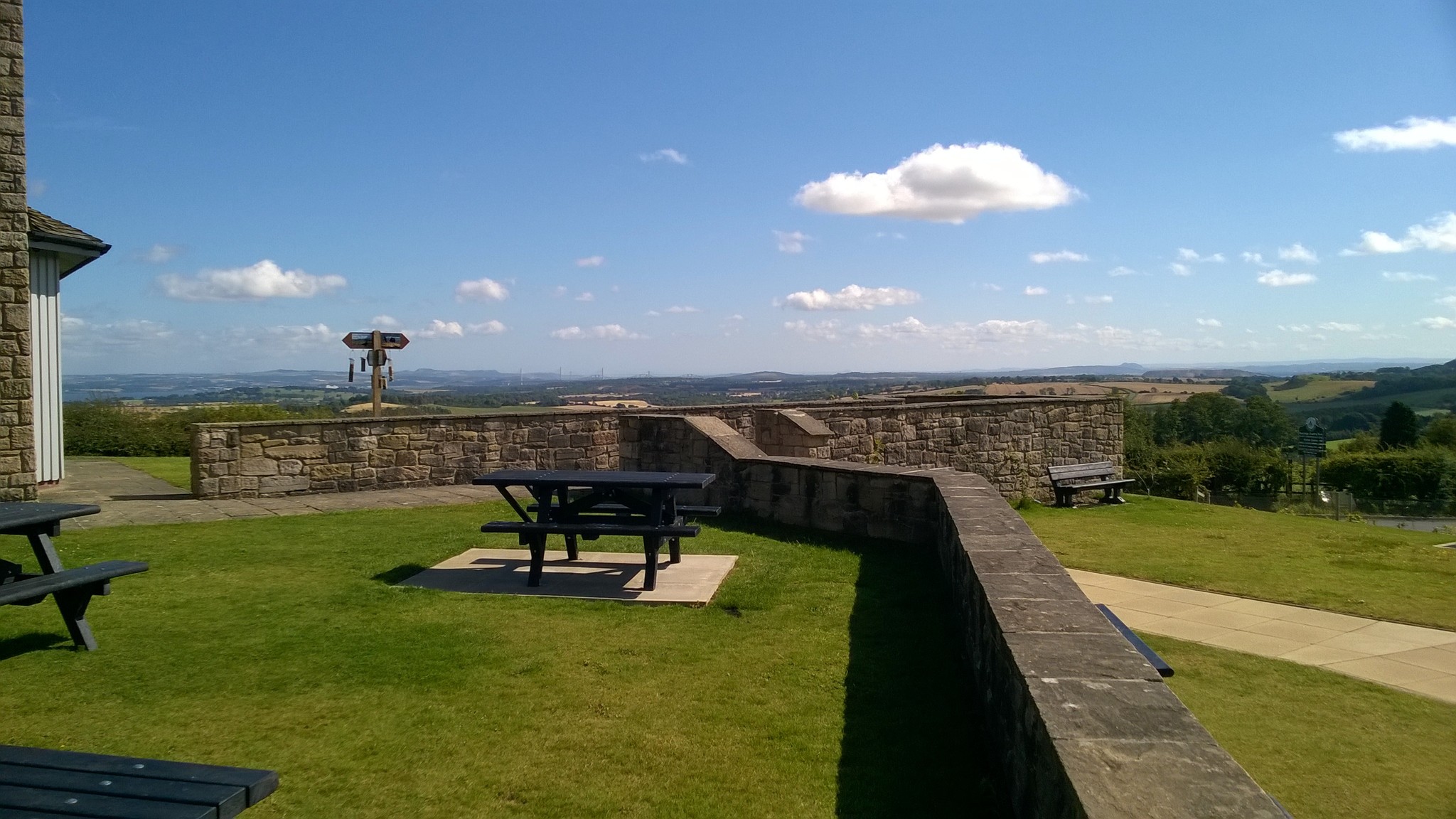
[471,469,716,591]
[0,743,276,819]
[0,501,101,652]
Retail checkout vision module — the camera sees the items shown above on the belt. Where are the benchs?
[480,503,722,551]
[1046,461,1136,508]
[1095,603,1174,678]
[0,560,150,621]
